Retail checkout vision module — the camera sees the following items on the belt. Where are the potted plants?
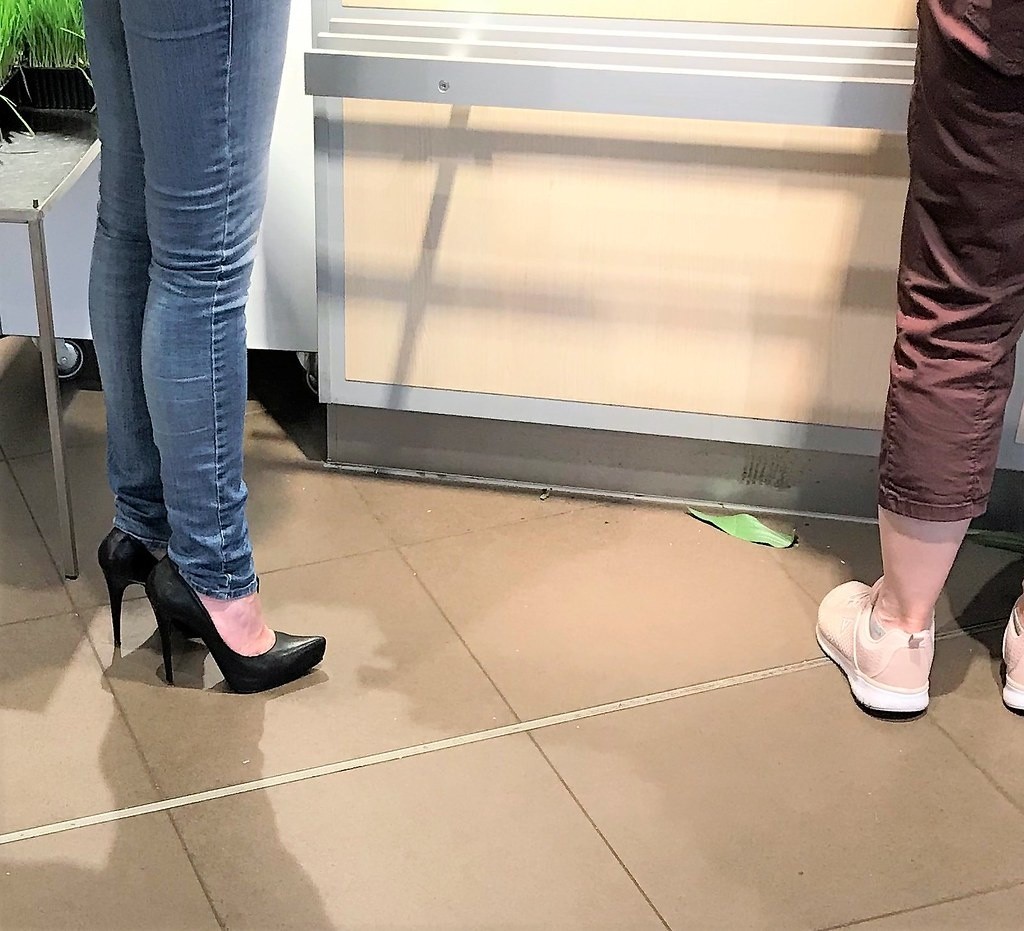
[0,0,97,137]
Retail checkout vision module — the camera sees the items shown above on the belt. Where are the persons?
[816,0,1024,713]
[81,1,325,694]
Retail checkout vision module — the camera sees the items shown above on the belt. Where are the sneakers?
[1002,581,1024,709]
[816,575,935,714]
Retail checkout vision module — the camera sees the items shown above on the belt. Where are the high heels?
[144,554,326,692]
[98,527,202,648]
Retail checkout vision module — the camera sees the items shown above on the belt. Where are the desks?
[0,120,100,581]
